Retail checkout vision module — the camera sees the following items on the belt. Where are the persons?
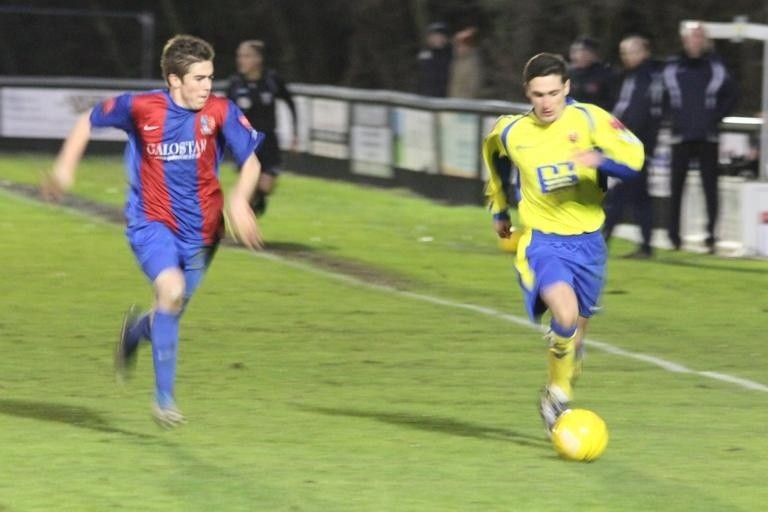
[225,38,300,218]
[661,21,735,258]
[567,34,611,106]
[414,21,490,100]
[481,53,647,440]
[48,34,267,431]
[597,34,669,263]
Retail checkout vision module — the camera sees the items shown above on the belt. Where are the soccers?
[553,409,608,460]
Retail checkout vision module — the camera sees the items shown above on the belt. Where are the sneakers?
[116,306,141,376]
[573,342,586,382]
[538,386,570,435]
[154,398,183,428]
[625,245,651,260]
